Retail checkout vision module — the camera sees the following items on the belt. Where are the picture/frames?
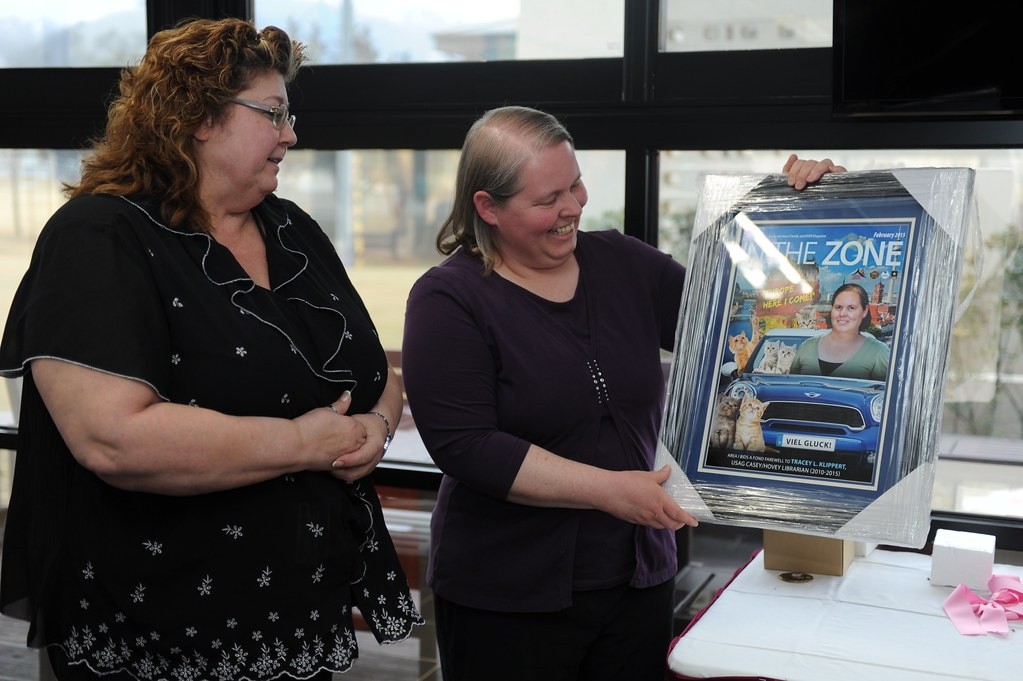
[651,166,974,547]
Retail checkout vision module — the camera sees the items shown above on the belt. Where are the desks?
[666,548,1023,681]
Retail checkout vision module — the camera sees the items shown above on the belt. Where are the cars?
[722,327,888,483]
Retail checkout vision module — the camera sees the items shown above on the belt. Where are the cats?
[776,342,797,374]
[728,317,758,377]
[711,391,738,448]
[734,393,779,453]
[753,339,780,374]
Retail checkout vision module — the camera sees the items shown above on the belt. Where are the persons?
[0,19,424,681]
[402,107,848,681]
[790,283,891,382]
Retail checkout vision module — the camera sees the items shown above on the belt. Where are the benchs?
[353,350,440,681]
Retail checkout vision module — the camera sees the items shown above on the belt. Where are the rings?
[329,405,337,412]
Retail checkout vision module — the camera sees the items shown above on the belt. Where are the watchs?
[369,411,391,458]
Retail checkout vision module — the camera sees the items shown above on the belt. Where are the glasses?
[229,96,295,131]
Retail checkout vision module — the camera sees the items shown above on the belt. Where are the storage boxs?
[763,528,852,577]
[930,528,996,591]
[857,543,880,555]
